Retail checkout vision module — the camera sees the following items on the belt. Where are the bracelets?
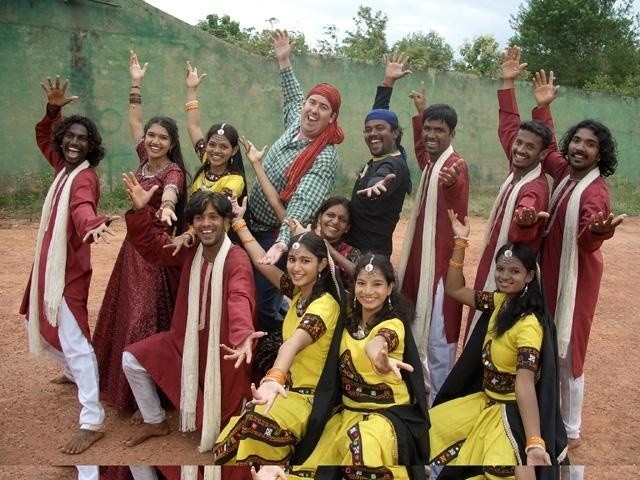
[448,258,466,267]
[160,200,177,213]
[127,93,142,104]
[522,436,545,453]
[185,100,200,111]
[260,369,288,387]
[130,85,141,88]
[240,237,259,245]
[233,219,247,232]
[451,234,471,241]
[182,232,196,248]
[372,355,388,375]
[452,242,469,249]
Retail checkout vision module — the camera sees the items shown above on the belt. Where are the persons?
[237,136,364,293]
[349,51,413,262]
[401,81,468,409]
[303,254,431,465]
[121,171,268,453]
[247,29,346,331]
[164,61,247,255]
[464,45,551,382]
[528,68,628,449]
[214,192,340,465]
[91,51,188,426]
[21,75,119,454]
[428,208,556,467]
